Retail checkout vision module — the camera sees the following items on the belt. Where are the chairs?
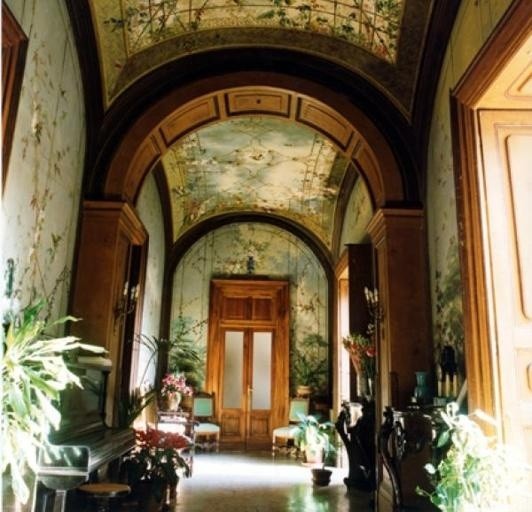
[190,396,220,453]
[271,396,310,461]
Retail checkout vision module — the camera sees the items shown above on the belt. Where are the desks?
[288,400,330,447]
[392,403,467,512]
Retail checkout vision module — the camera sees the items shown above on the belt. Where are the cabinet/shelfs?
[156,410,189,455]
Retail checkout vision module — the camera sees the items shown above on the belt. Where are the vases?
[133,477,168,512]
[355,375,375,398]
[412,371,429,400]
[168,390,181,413]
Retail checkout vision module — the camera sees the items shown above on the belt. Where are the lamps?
[113,280,141,334]
[364,286,383,323]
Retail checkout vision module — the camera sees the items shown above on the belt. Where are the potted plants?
[306,413,342,486]
[288,331,330,397]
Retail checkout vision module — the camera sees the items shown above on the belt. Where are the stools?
[76,483,131,512]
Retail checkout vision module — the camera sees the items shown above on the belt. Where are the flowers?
[123,428,194,485]
[342,324,375,378]
[160,369,193,399]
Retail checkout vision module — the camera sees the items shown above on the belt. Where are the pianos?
[2,361,136,512]
